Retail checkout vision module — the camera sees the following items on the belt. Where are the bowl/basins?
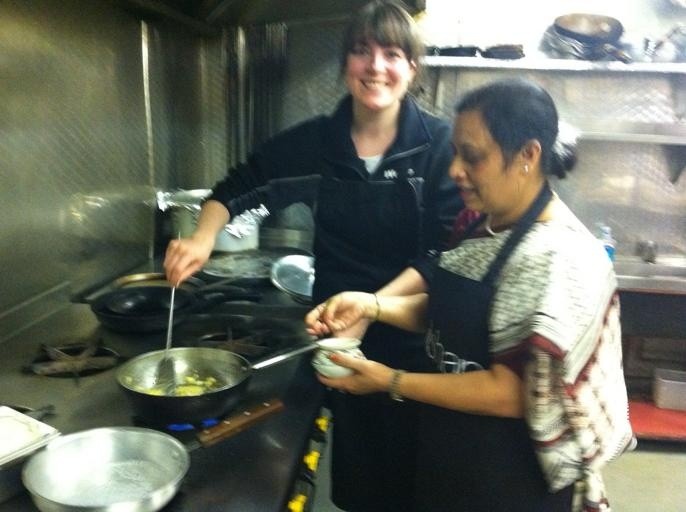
[157,202,262,254]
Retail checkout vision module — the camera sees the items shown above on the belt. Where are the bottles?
[597,224,617,266]
[311,339,367,380]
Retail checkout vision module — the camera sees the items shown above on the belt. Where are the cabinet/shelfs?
[414,47,686,152]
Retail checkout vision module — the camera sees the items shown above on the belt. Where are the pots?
[93,283,263,334]
[108,274,245,289]
[22,397,285,512]
[114,339,322,423]
[430,42,526,61]
[551,10,634,68]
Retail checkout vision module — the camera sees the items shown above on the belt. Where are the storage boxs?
[649,366,686,414]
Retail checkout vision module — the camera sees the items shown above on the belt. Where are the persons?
[162,0,485,511]
[303,79,640,512]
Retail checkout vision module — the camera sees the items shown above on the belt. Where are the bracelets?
[386,367,404,405]
[372,292,382,324]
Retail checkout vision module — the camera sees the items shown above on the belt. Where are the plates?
[268,252,314,301]
